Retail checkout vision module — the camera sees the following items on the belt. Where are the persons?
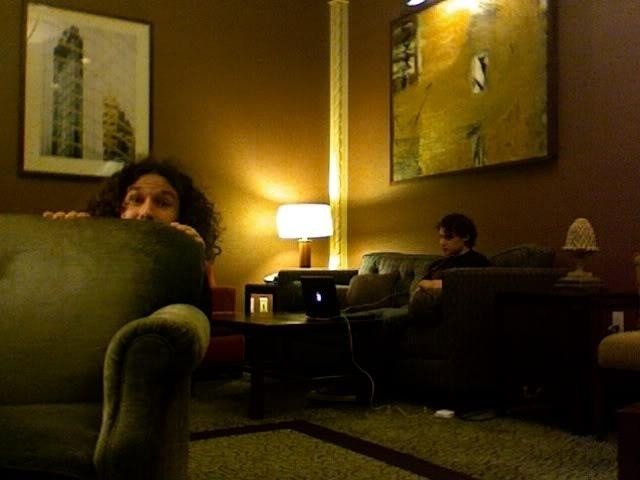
[41,156,220,313]
[417,213,489,288]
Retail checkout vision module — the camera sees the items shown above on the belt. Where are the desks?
[518,287,633,436]
[212,310,384,416]
[244,282,305,317]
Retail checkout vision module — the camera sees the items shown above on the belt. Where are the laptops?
[299,276,369,321]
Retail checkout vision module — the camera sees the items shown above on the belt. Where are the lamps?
[274,203,335,269]
[559,217,600,283]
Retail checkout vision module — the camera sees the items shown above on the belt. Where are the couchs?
[277,244,567,412]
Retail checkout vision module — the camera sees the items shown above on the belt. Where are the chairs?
[597,326,640,479]
[0,209,218,479]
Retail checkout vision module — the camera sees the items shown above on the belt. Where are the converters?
[434,410,455,418]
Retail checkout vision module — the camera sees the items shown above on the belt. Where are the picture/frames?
[16,2,156,185]
[387,0,561,186]
